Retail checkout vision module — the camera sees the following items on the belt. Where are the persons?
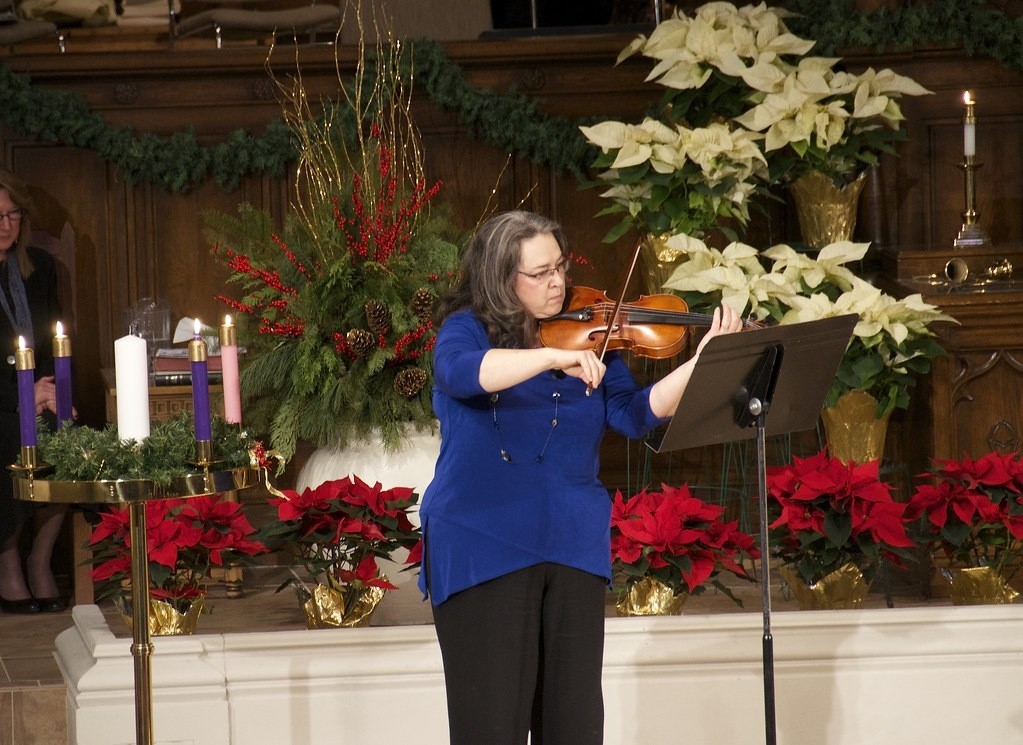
[0,169,91,614]
[417,210,743,745]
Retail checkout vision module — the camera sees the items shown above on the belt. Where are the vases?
[106,574,390,635]
[635,231,712,295]
[779,559,877,609]
[938,566,1021,606]
[782,165,869,266]
[616,576,688,617]
[817,390,895,464]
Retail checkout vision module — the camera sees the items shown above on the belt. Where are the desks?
[10,464,263,745]
[90,369,222,432]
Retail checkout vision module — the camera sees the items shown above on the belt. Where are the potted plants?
[196,0,602,588]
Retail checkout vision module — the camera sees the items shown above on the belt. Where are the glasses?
[0,208,25,222]
[516,255,570,282]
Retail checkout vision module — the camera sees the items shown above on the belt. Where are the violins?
[538,285,772,360]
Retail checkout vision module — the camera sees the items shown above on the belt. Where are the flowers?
[577,0,961,420]
[609,443,1023,575]
[78,473,423,601]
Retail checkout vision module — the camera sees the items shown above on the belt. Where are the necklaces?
[490,374,560,463]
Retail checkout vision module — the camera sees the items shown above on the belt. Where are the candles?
[52,336,73,433]
[221,324,241,424]
[15,349,38,467]
[188,337,214,462]
[965,102,977,164]
[114,334,151,448]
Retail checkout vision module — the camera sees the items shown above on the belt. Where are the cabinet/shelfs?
[8,34,1023,566]
